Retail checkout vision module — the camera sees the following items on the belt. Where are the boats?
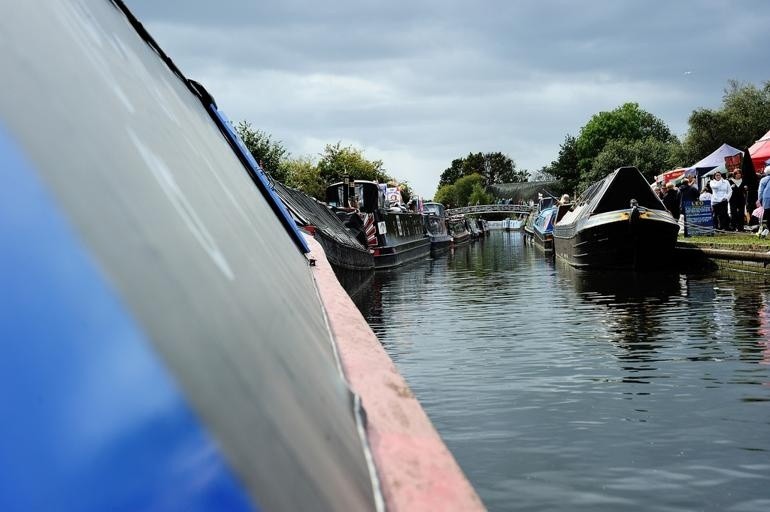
[259,165,490,272]
[524,166,680,269]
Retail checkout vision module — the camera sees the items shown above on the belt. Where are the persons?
[654,166,770,237]
[444,197,534,210]
